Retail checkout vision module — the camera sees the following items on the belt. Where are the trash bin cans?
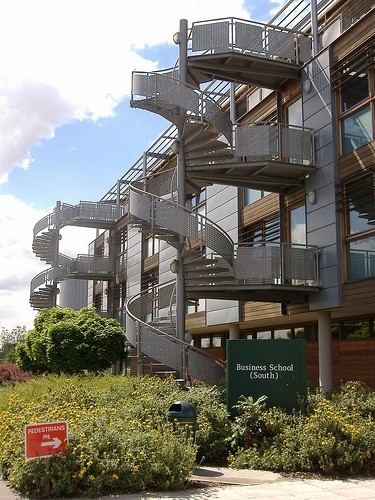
[165,400,197,445]
